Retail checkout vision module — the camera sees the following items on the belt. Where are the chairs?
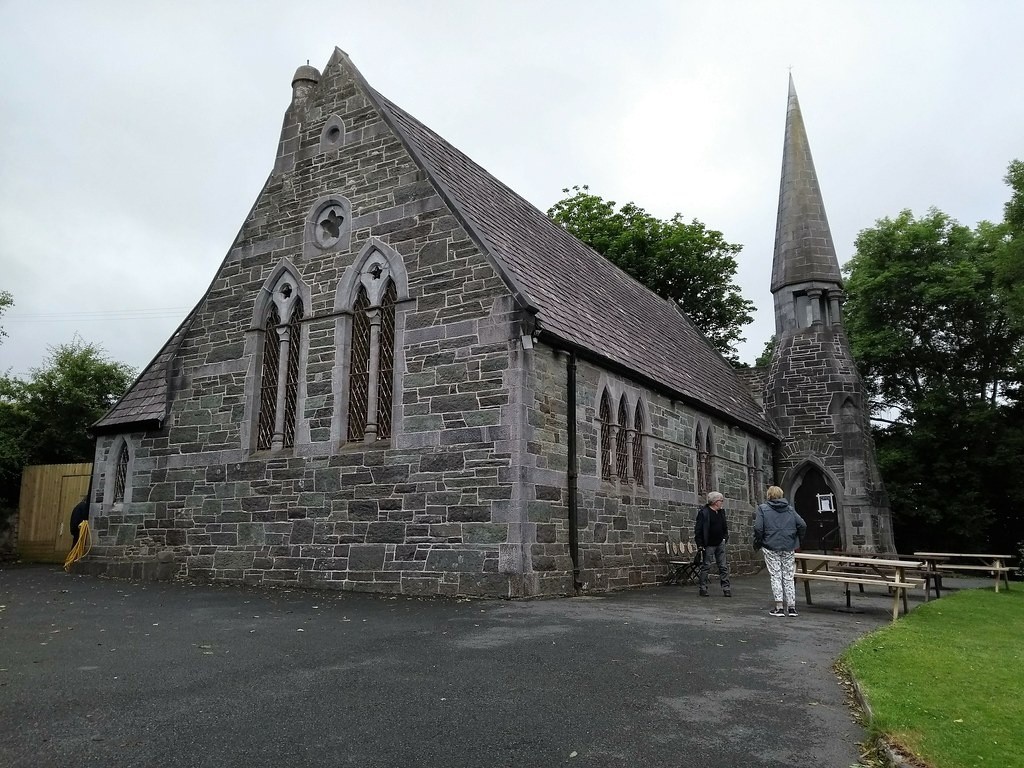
[665,540,711,588]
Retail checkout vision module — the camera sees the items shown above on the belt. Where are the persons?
[70,495,88,550]
[694,491,732,596]
[755,486,807,616]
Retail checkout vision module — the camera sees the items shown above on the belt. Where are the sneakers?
[769,609,785,616]
[788,608,798,617]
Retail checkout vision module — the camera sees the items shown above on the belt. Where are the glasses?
[717,499,723,502]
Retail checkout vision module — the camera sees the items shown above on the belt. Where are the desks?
[793,552,922,625]
[834,552,951,602]
[914,553,1017,593]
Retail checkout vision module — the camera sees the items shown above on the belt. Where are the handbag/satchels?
[693,550,705,566]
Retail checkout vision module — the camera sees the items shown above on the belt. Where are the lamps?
[554,350,570,356]
[521,333,535,350]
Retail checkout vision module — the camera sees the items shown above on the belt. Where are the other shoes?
[724,590,731,596]
[700,590,709,596]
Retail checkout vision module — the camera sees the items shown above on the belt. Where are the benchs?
[794,566,944,589]
[920,564,1020,571]
